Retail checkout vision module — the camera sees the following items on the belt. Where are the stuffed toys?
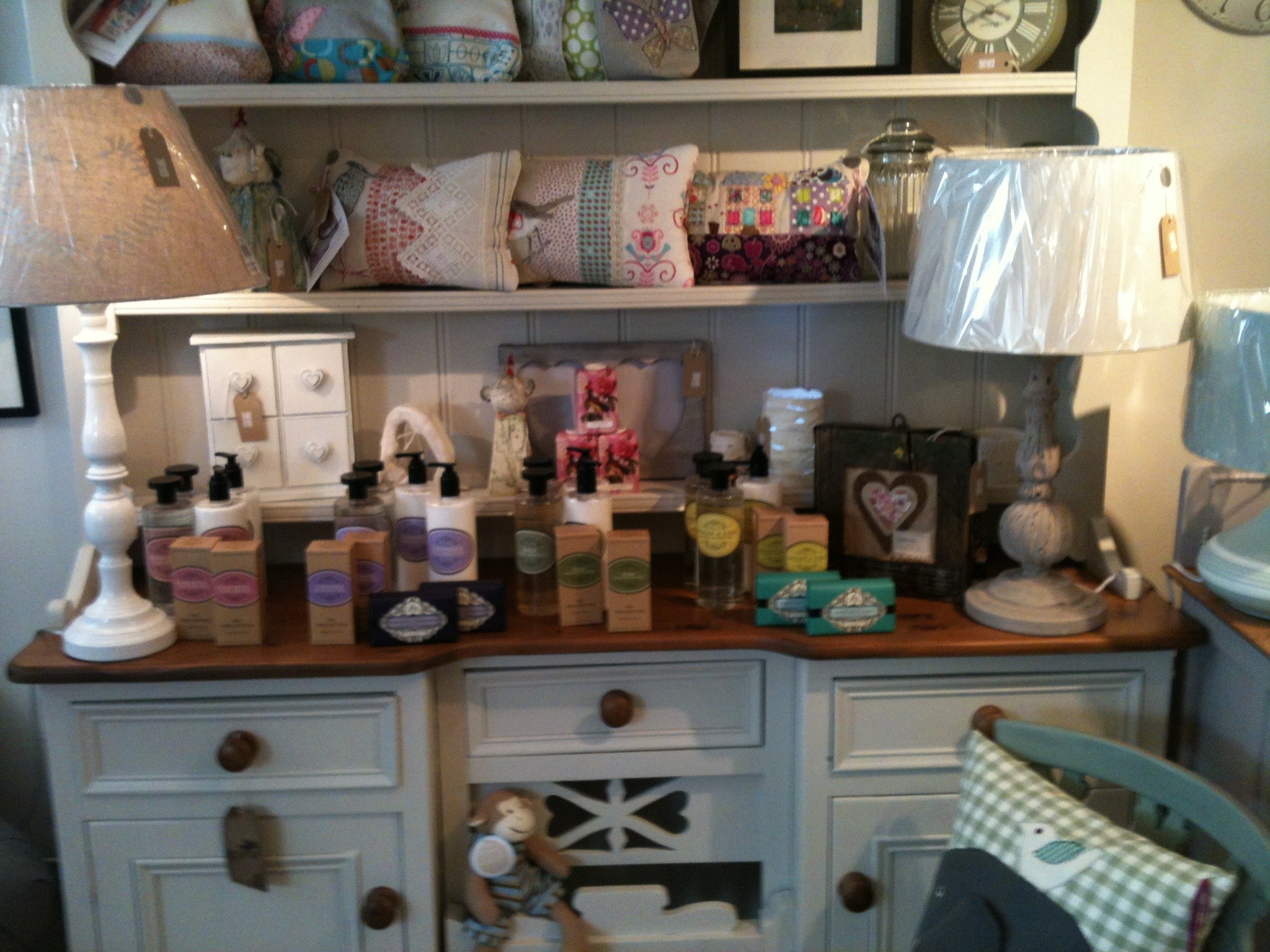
[459,790,592,952]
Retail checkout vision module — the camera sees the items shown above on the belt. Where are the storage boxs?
[749,505,898,636]
[166,533,265,646]
[554,522,653,634]
[303,532,395,648]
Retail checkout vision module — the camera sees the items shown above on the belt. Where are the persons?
[211,110,307,293]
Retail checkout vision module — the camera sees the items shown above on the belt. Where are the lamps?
[0,86,271,662]
[897,146,1198,638]
[1183,292,1270,619]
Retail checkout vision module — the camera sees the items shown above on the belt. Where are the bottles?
[862,118,934,279]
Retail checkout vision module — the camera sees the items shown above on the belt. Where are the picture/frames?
[815,417,977,603]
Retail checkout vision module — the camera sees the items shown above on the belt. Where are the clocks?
[932,1,1068,74]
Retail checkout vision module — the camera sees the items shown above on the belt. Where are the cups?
[973,427,1025,503]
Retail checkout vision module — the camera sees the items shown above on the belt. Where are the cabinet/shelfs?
[22,0,1147,630]
[8,561,1211,952]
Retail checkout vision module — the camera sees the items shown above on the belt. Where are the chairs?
[993,713,1270,951]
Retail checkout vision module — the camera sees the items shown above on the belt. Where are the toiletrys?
[303,450,507,651]
[679,450,898,638]
[139,447,269,647]
[515,444,657,633]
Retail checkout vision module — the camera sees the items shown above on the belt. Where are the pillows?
[315,145,524,293]
[683,155,886,287]
[902,732,1239,952]
[506,143,699,290]
[106,0,700,86]
